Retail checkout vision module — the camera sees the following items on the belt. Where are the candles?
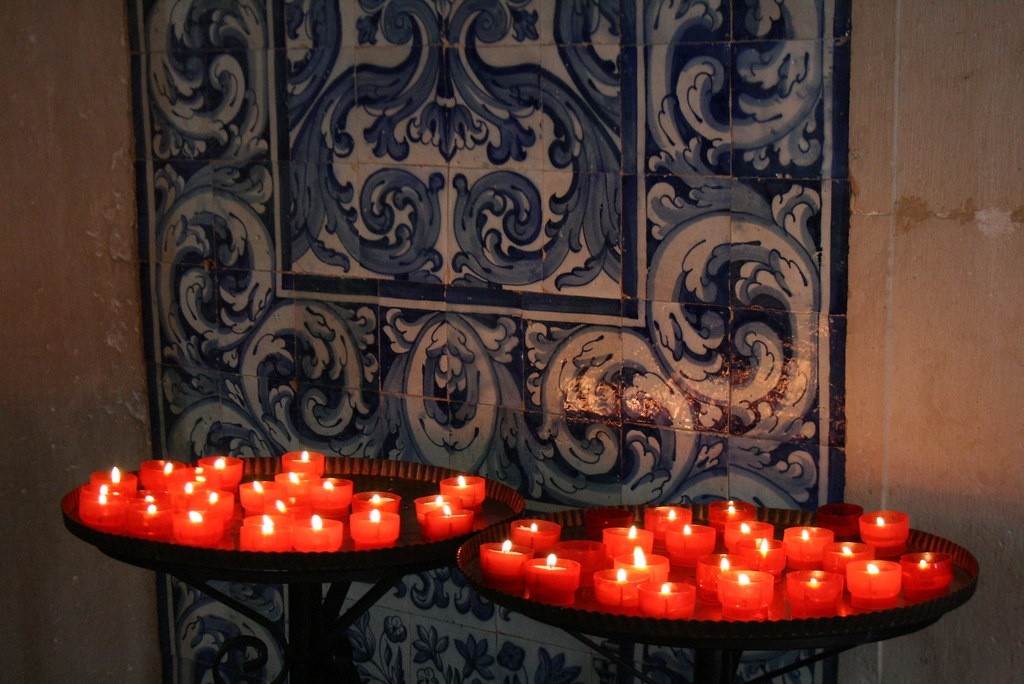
[67,449,976,633]
